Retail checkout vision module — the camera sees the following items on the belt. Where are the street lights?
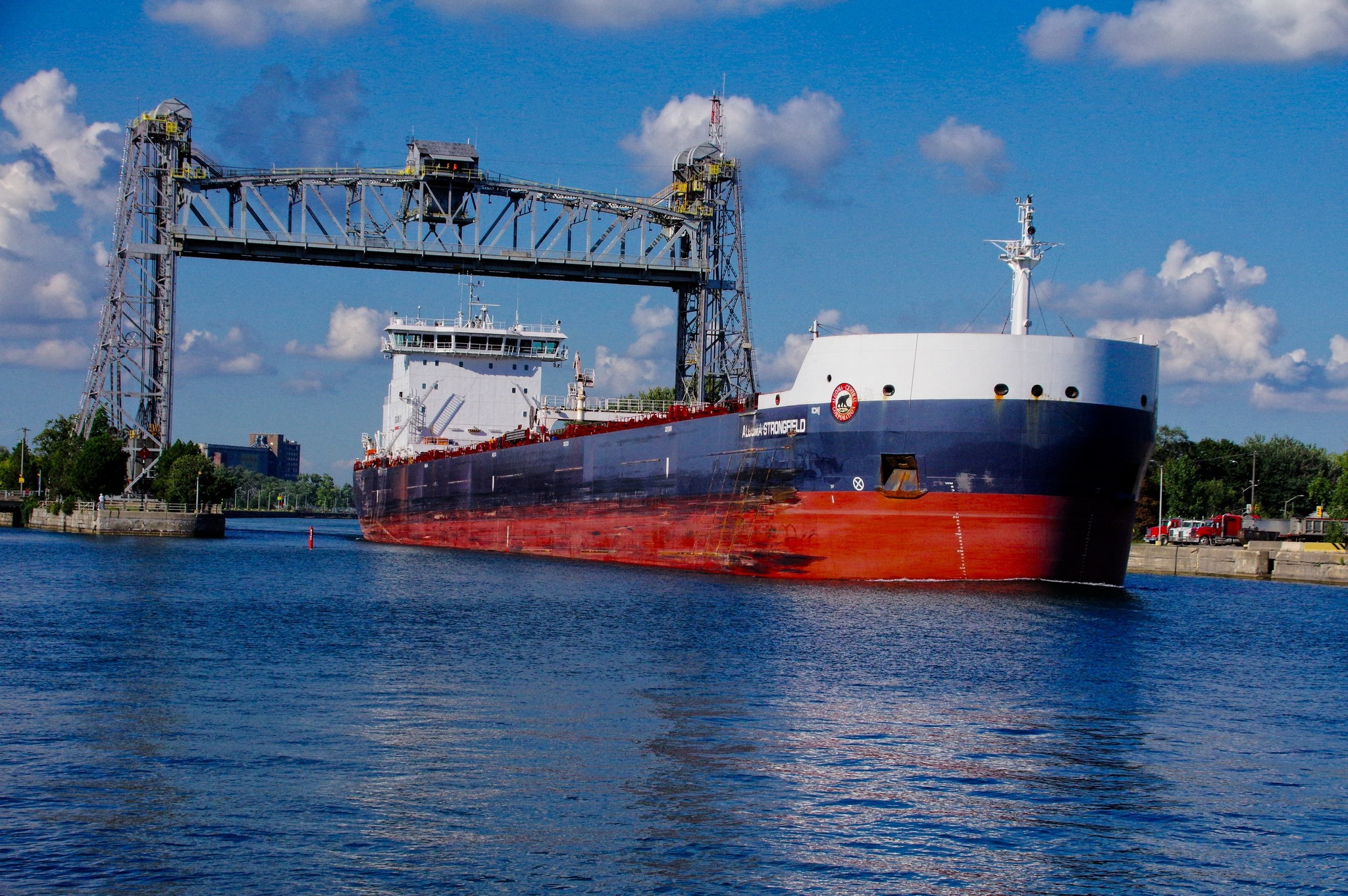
[269,491,275,510]
[258,490,265,509]
[196,471,201,510]
[287,493,292,507]
[1242,485,1257,516]
[235,487,242,508]
[278,492,284,509]
[247,489,254,508]
[296,494,301,509]
[1284,495,1304,518]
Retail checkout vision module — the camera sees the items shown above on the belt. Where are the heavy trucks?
[1144,515,1290,546]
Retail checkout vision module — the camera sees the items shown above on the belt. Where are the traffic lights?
[1317,507,1322,517]
[1254,505,1258,514]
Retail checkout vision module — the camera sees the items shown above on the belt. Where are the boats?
[352,194,1159,588]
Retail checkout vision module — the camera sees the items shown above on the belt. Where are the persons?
[502,433,505,437]
[475,316,482,324]
[725,401,731,414]
[490,436,495,450]
[1167,518,1172,533]
[25,488,30,496]
[99,493,105,502]
